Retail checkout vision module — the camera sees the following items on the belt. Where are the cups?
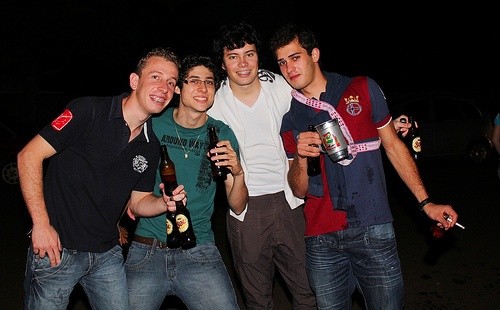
[315,118,349,164]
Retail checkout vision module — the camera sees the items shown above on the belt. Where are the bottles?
[166,209,180,250]
[170,190,197,251]
[430,212,449,242]
[398,118,425,167]
[208,127,227,182]
[159,144,178,195]
[307,124,322,177]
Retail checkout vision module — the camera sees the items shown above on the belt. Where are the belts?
[132,233,168,248]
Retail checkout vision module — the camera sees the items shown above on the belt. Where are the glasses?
[183,79,215,88]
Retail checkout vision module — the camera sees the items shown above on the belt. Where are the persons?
[491,113,500,154]
[266,26,458,310]
[123,57,249,310]
[206,21,418,310]
[17,48,188,310]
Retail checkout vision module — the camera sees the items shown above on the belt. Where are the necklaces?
[173,129,199,159]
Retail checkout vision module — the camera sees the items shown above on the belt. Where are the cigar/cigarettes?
[443,216,464,229]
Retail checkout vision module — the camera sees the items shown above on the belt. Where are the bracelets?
[419,197,432,208]
[233,167,245,175]
